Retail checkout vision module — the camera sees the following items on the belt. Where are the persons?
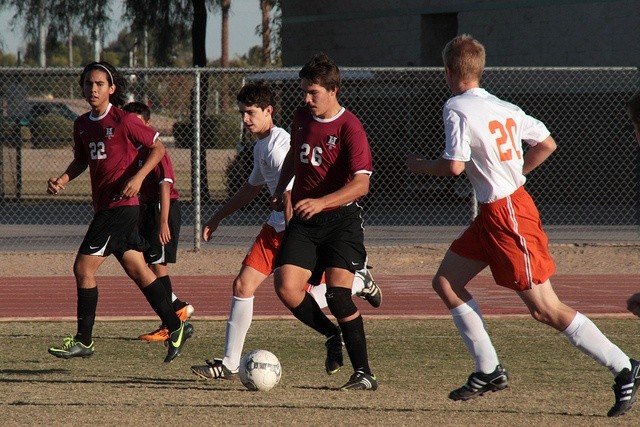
[272,52,381,393]
[44,62,194,365]
[192,82,382,381]
[403,33,639,417]
[119,101,195,347]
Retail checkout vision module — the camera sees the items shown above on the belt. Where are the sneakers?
[325,326,344,374]
[139,324,170,342]
[47,335,94,358]
[607,358,640,416]
[190,357,239,381]
[175,298,195,320]
[356,268,382,307]
[338,369,377,391]
[449,364,509,400]
[163,319,194,361]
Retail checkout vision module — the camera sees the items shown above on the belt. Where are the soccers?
[239,350,281,391]
